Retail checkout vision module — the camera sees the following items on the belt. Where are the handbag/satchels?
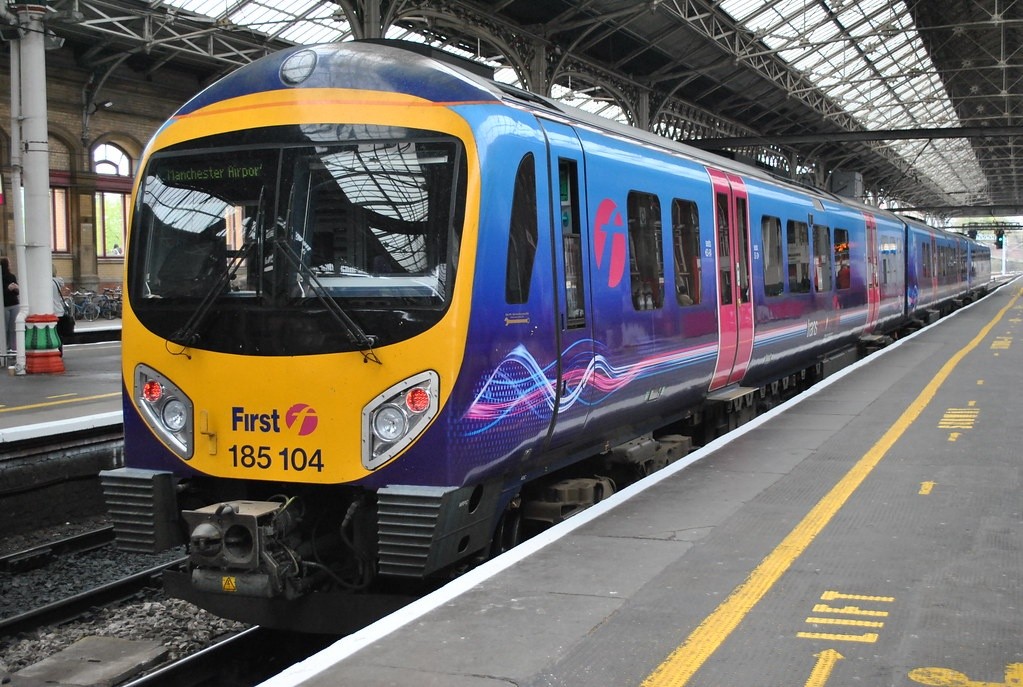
[63,313,75,333]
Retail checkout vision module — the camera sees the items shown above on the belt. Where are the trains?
[97,42,995,636]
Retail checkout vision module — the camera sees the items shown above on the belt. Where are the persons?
[0,255,21,360]
[111,244,124,256]
[801,273,811,293]
[678,286,694,307]
[51,263,66,358]
[837,263,851,289]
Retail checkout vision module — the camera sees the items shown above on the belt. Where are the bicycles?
[63,285,123,322]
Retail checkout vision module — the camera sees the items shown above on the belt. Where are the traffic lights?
[996,233,1003,249]
